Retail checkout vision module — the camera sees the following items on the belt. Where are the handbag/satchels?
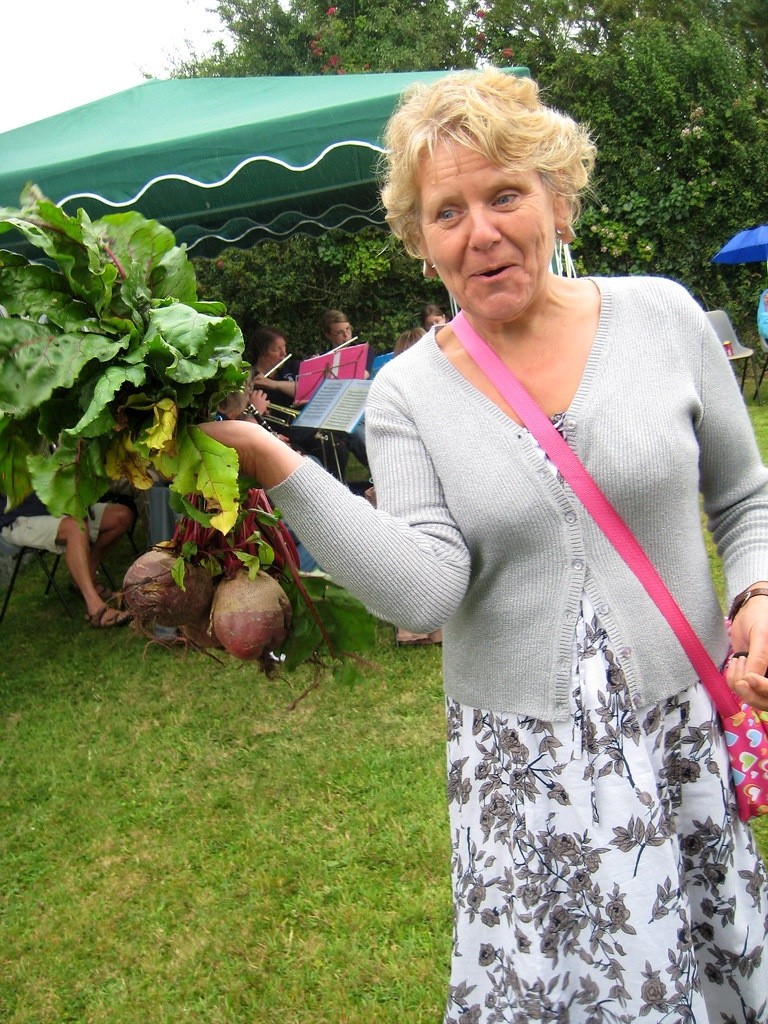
[716,615,768,818]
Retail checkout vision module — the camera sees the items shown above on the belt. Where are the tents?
[0,66,578,279]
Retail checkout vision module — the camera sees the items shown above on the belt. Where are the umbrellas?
[712,224,768,273]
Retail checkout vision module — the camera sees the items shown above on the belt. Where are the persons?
[310,310,369,470]
[224,377,326,578]
[146,459,187,639]
[757,289,768,342]
[369,328,428,508]
[422,305,450,333]
[0,443,130,626]
[244,328,350,479]
[108,69,768,1024]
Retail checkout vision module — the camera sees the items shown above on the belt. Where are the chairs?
[705,310,768,405]
[0,491,139,620]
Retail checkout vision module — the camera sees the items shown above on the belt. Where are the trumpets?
[242,401,331,442]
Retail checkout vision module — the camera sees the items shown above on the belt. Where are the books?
[293,378,373,432]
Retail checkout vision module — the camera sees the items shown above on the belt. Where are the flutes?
[246,400,278,438]
[264,353,292,378]
[322,336,359,356]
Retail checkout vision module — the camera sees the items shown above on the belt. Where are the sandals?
[85,604,133,628]
[67,579,117,601]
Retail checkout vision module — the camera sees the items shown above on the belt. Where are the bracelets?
[728,588,768,623]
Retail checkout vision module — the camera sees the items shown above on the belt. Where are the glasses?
[331,326,354,339]
